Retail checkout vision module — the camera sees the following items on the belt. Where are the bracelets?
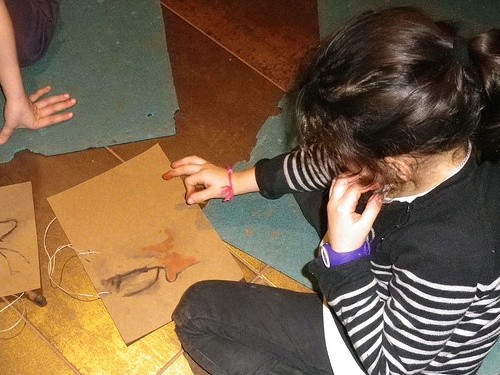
[219,165,234,202]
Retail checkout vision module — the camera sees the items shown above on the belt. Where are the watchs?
[320,239,371,268]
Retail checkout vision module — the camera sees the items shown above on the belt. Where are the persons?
[0,0,77,145]
[162,6,500,375]
